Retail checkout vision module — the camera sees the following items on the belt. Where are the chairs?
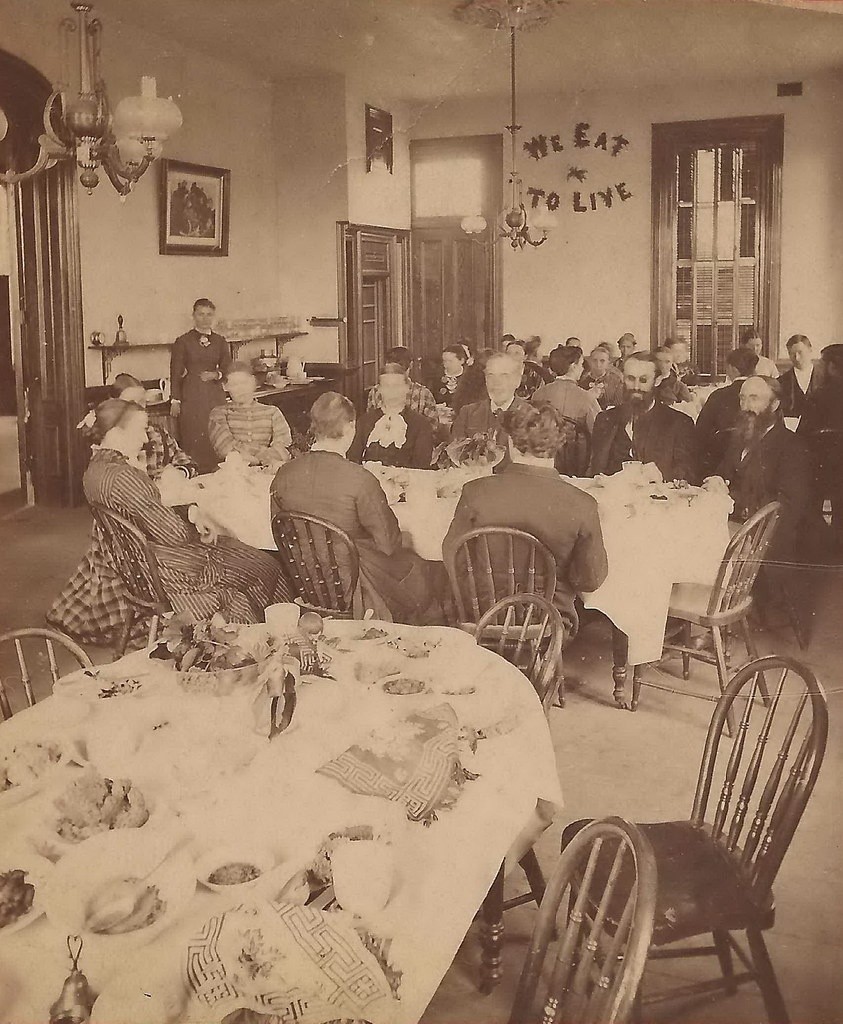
[271,510,360,620]
[0,628,94,718]
[561,655,829,1024]
[472,592,569,943]
[623,499,786,737]
[507,815,658,1024]
[90,501,173,660]
[453,525,569,710]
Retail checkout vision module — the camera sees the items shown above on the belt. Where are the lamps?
[0,0,184,205]
[453,0,570,249]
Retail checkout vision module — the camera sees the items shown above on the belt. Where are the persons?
[169,179,218,238]
[440,398,609,649]
[89,334,842,622]
[168,298,231,476]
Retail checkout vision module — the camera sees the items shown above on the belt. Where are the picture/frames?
[160,157,232,258]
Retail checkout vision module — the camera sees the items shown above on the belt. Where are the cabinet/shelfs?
[87,332,348,521]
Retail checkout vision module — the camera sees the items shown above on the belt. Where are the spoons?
[86,827,190,930]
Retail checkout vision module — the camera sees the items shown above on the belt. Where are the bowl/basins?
[44,827,193,945]
[342,620,394,647]
[196,845,276,894]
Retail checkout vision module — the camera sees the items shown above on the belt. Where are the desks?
[169,461,734,709]
[0,623,556,1024]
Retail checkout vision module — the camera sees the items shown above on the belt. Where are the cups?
[264,602,300,632]
[332,841,393,915]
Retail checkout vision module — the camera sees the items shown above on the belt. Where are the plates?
[376,673,430,697]
[1,737,72,808]
[0,853,54,937]
[51,664,155,706]
